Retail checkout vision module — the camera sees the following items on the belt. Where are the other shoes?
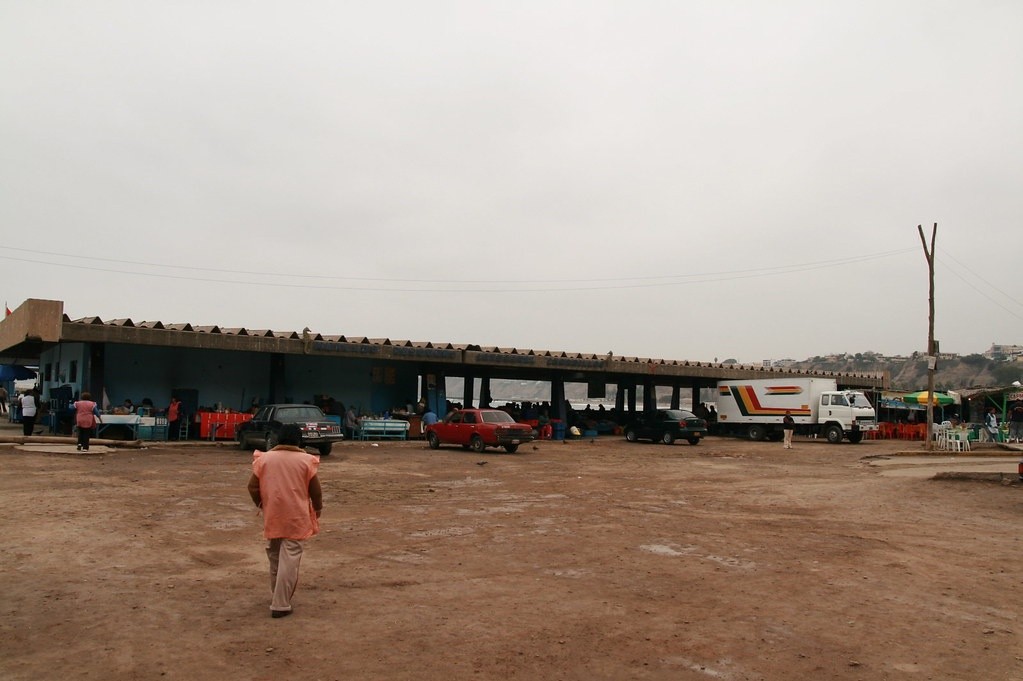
[272,609,293,618]
[83,449,88,452]
[77,444,81,450]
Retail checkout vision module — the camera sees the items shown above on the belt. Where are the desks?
[352,419,411,441]
[193,411,255,441]
[94,414,145,440]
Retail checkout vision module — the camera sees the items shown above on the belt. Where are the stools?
[352,426,361,441]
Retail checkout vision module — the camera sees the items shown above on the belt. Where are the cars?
[624,409,708,445]
[424,409,534,453]
[235,404,343,455]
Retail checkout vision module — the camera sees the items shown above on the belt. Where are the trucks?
[716,377,880,444]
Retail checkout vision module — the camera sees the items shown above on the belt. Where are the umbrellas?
[903,391,954,406]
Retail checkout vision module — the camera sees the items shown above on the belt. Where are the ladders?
[178,414,189,439]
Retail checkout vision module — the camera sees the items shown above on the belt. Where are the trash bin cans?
[8,406,23,420]
[552,423,565,440]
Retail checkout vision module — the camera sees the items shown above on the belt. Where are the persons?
[585,404,591,410]
[346,406,361,441]
[954,413,961,425]
[167,397,182,440]
[21,389,38,436]
[599,404,605,411]
[74,393,103,452]
[985,407,1000,442]
[783,411,794,449]
[0,384,7,413]
[247,424,323,618]
[694,402,717,418]
[121,399,135,414]
[1008,399,1023,443]
[422,409,438,426]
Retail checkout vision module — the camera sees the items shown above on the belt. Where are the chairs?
[864,420,1023,452]
